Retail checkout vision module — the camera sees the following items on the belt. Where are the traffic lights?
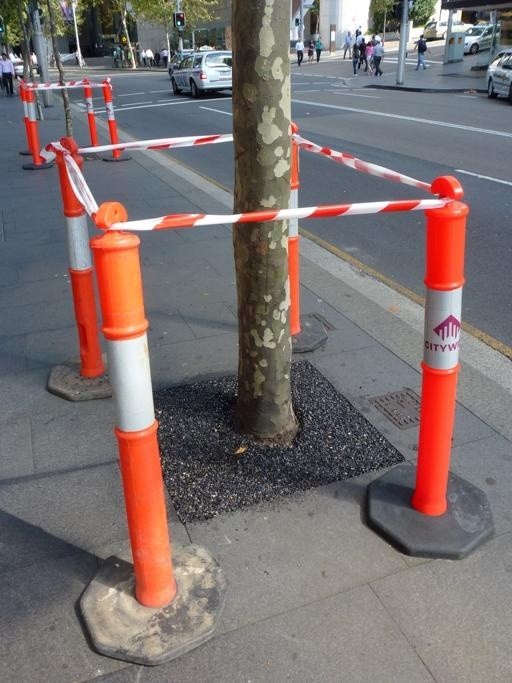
[121,34,127,46]
[174,12,185,28]
[0,15,5,33]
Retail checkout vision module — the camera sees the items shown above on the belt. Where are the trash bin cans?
[447,32,466,63]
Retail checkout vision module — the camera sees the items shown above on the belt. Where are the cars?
[171,49,233,97]
[11,58,30,77]
[462,23,500,54]
[168,50,195,79]
[486,48,512,104]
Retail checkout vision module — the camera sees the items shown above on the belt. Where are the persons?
[0,53,17,97]
[108,43,182,70]
[74,50,80,65]
[294,24,388,77]
[29,51,37,65]
[412,34,432,72]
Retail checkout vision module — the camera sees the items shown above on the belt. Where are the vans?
[423,19,474,40]
